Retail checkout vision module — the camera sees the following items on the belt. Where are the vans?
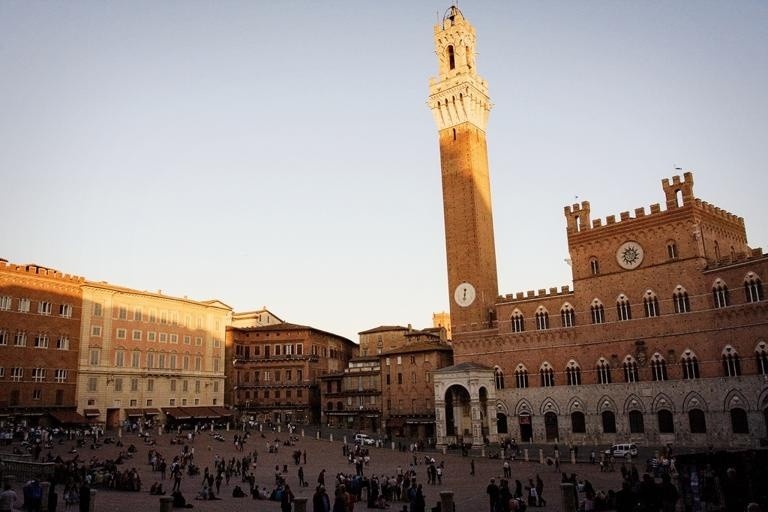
[604,443,638,458]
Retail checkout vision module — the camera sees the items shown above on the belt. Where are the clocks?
[615,240,644,271]
[454,282,477,308]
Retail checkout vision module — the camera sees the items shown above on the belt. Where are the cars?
[354,433,375,446]
